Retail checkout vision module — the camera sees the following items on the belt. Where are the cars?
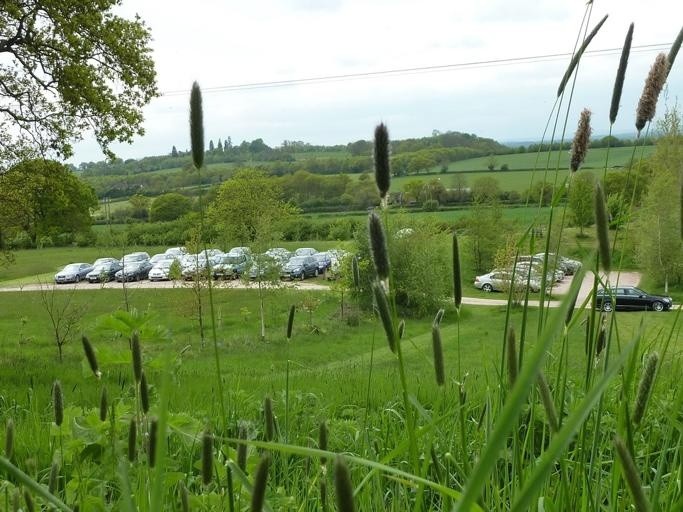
[474,252,582,293]
[54,262,93,284]
[86,247,332,280]
[591,286,672,311]
